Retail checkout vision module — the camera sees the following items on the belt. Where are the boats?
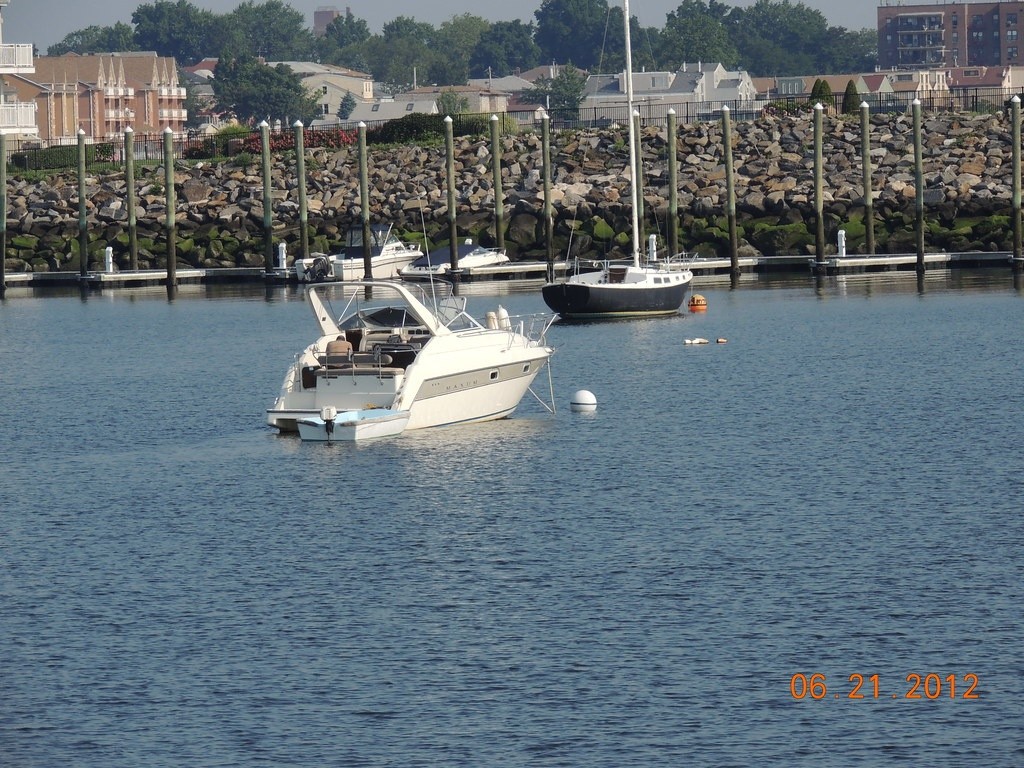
[295,221,424,282]
[266,280,561,430]
[396,237,511,283]
[296,405,411,441]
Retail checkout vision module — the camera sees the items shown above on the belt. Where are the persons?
[326,335,356,368]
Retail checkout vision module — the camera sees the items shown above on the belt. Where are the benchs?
[314,351,403,385]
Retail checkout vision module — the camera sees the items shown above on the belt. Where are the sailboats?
[543,0,699,317]
